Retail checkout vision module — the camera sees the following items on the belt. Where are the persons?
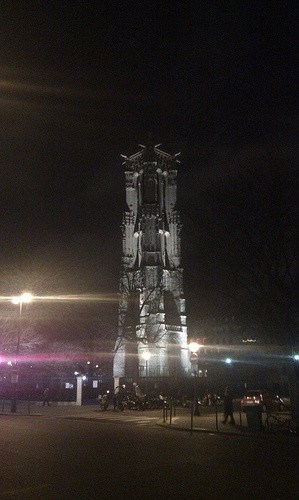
[221,386,236,425]
[42,388,49,406]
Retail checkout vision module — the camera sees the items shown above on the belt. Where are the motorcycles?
[97,390,110,412]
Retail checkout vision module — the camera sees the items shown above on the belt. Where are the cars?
[240,389,285,413]
[141,391,170,411]
[195,391,225,407]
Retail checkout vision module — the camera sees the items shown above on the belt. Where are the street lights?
[11,292,32,413]
[189,342,200,416]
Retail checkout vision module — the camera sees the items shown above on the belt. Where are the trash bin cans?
[245,405,265,432]
